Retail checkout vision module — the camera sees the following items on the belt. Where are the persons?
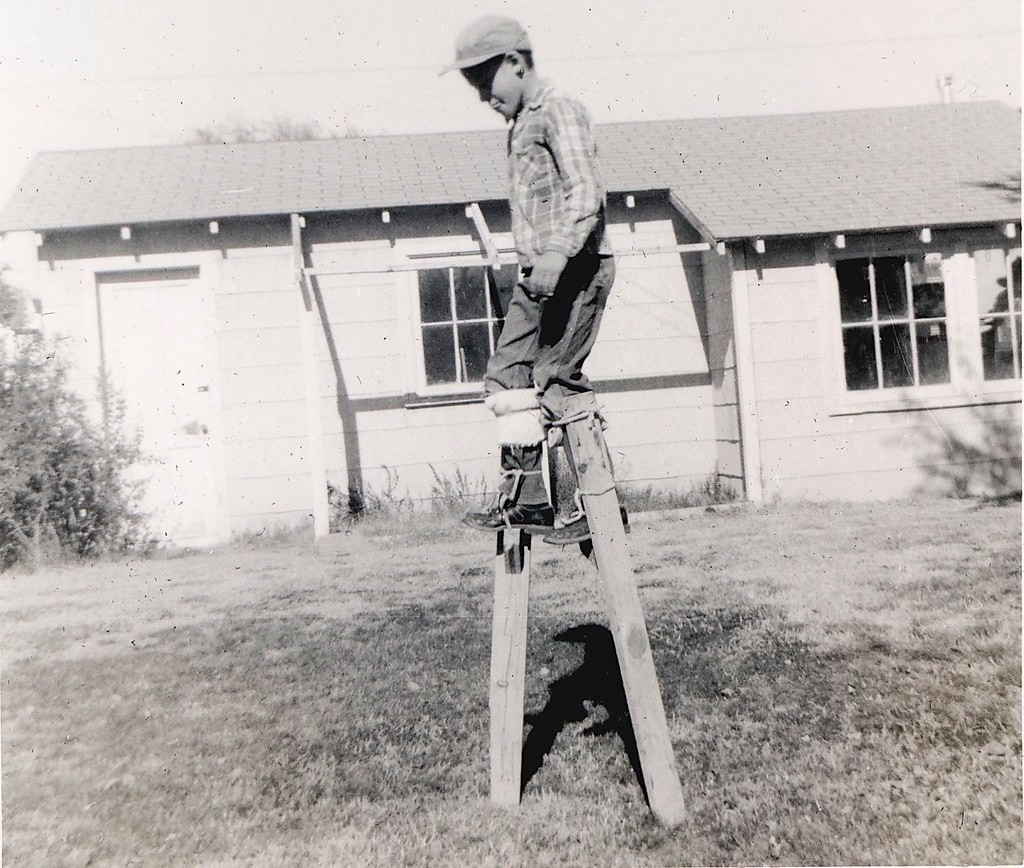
[435,18,632,544]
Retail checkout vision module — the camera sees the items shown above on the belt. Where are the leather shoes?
[543,506,630,543]
[463,497,554,535]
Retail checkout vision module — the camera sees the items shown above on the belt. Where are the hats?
[437,15,532,76]
